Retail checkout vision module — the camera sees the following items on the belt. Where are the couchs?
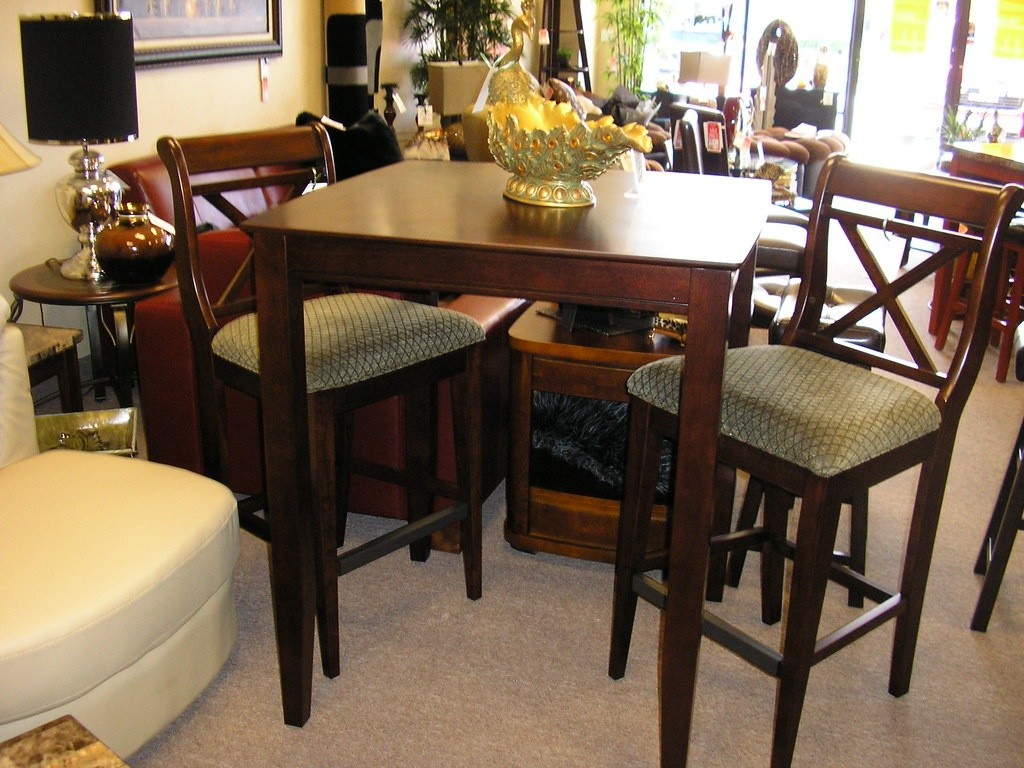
[0,297,240,761]
[103,151,531,520]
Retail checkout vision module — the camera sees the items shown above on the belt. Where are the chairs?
[668,102,814,277]
[154,119,487,682]
[608,152,1021,768]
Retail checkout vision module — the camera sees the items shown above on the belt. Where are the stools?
[934,224,1024,384]
[17,324,87,412]
[729,269,891,606]
[744,126,851,202]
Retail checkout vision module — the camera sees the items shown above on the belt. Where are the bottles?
[95,202,177,288]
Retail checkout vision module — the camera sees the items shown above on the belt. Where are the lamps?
[20,11,139,278]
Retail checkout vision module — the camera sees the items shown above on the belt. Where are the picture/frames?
[94,0,284,68]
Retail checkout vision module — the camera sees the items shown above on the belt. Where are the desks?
[9,255,181,407]
[504,296,678,565]
[239,160,777,767]
[928,141,1024,338]
